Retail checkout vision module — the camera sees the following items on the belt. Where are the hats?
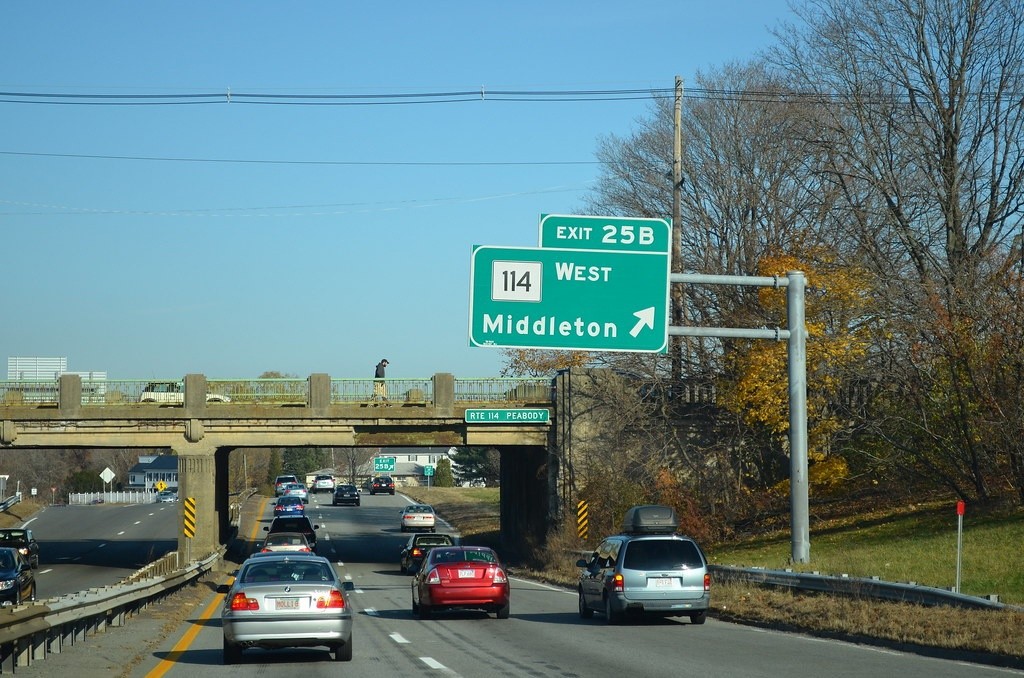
[382,358,389,364]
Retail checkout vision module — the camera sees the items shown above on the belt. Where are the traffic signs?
[217,557,356,662]
[374,458,395,472]
[467,212,673,356]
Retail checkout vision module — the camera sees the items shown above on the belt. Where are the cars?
[368,475,396,495]
[312,476,336,494]
[272,475,310,503]
[156,487,179,503]
[399,534,456,575]
[0,528,39,606]
[140,381,231,408]
[411,546,510,620]
[250,515,319,558]
[399,504,436,533]
[89,499,104,505]
[270,496,305,516]
[332,484,360,506]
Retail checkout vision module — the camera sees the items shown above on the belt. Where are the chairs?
[303,568,323,581]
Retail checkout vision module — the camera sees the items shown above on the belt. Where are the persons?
[373,359,392,407]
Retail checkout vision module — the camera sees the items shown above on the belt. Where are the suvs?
[576,504,710,625]
